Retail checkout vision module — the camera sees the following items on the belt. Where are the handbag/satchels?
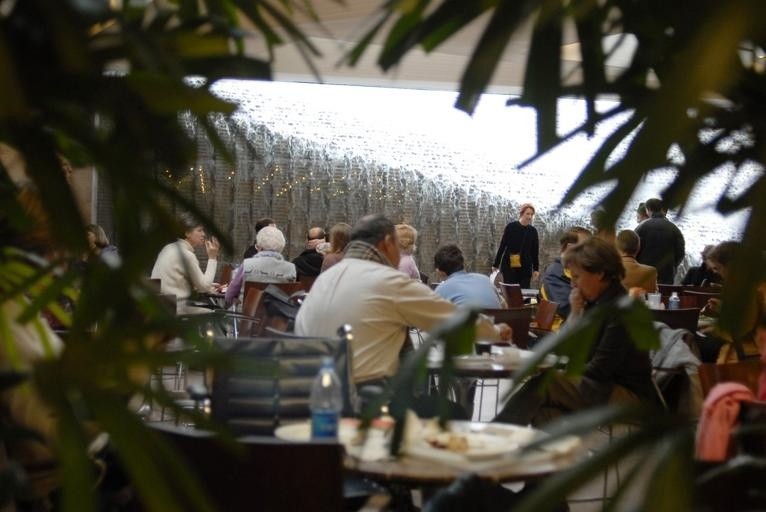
[510,254,522,268]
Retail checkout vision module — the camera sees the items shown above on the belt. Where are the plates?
[447,419,545,459]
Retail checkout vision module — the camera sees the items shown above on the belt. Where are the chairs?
[130,272,766,512]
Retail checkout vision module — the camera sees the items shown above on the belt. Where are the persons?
[493,204,540,303]
[78,223,122,273]
[432,245,509,309]
[150,213,221,315]
[293,215,513,419]
[491,237,657,512]
[222,214,422,332]
[538,197,766,365]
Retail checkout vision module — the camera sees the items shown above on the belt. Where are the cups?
[476,337,492,356]
[648,293,661,309]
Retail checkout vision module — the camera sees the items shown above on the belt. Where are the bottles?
[307,357,344,438]
[668,291,681,309]
